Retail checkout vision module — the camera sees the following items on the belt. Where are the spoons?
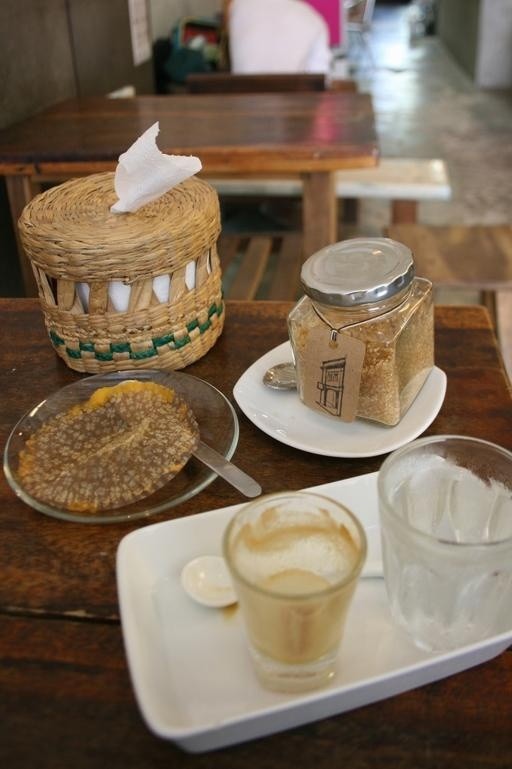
[262,361,297,391]
[182,555,383,608]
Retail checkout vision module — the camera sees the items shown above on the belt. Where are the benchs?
[205,155,454,225]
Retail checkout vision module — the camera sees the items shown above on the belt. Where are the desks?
[0,91,378,298]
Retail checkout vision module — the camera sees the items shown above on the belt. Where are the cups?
[222,489,367,695]
[378,435,512,654]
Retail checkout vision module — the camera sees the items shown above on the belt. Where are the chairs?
[185,74,325,92]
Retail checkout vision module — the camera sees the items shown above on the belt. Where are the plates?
[3,368,240,521]
[235,338,449,458]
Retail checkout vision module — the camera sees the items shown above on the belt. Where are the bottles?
[289,237,435,426]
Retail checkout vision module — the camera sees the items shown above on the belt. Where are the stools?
[381,221,512,337]
